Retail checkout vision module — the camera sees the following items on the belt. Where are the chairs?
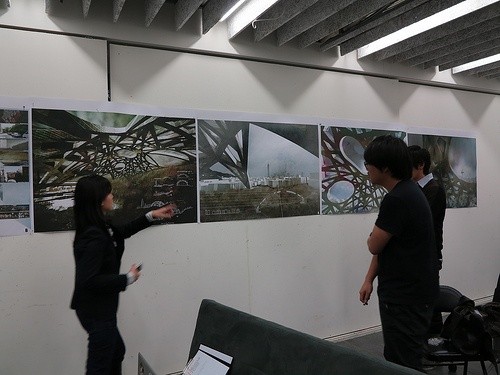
[423,285,488,375]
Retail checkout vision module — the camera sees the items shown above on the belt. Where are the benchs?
[183,299,426,375]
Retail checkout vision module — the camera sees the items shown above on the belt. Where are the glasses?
[364,161,370,171]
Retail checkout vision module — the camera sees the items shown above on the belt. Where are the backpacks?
[441,310,490,362]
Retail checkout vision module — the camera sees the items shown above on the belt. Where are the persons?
[359,135,435,373]
[407,145,458,375]
[70,174,177,375]
